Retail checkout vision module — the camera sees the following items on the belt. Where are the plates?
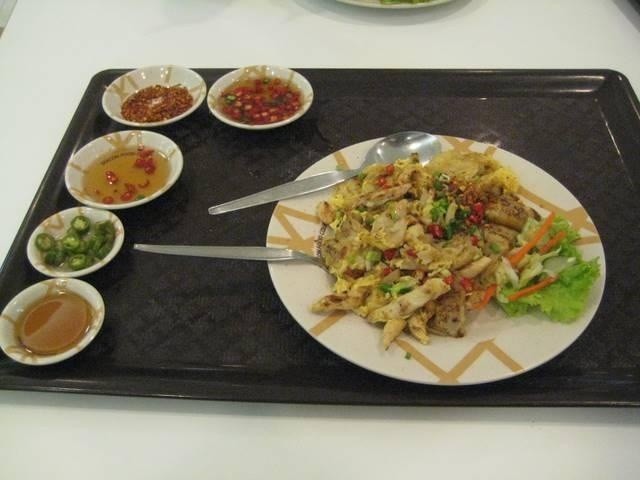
[102,67,206,126]
[1,278,108,367]
[265,133,606,387]
[64,130,186,210]
[207,67,315,128]
[25,206,124,278]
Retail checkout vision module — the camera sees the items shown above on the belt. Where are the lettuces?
[491,213,601,324]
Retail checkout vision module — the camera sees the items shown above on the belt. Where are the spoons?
[208,131,441,216]
